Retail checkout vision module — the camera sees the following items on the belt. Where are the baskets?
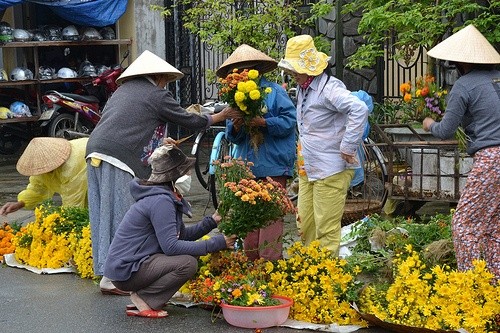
[340,199,382,228]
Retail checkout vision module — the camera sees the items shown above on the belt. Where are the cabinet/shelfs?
[0,39,131,154]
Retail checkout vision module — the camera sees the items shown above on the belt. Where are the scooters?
[38,49,132,140]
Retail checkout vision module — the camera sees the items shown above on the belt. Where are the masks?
[174,174,191,194]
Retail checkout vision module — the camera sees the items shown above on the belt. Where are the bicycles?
[285,137,392,209]
[192,70,298,217]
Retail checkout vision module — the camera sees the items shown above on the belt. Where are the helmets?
[0,106,14,120]
[0,21,115,42]
[0,62,113,82]
[10,101,32,117]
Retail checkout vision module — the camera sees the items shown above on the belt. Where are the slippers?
[100,287,133,295]
[126,303,166,310]
[125,309,170,318]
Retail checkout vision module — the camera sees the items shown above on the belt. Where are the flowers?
[217,68,272,133]
[400,76,476,148]
[211,154,297,240]
[1,197,500,333]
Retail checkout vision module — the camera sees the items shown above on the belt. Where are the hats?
[426,23,500,64]
[115,50,184,85]
[216,44,279,79]
[277,34,332,76]
[16,137,71,176]
[147,144,197,182]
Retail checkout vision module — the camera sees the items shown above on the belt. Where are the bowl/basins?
[220,295,293,328]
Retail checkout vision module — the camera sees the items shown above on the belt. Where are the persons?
[214,43,297,261]
[102,145,236,319]
[85,49,234,296]
[423,25,500,286]
[0,136,90,217]
[275,35,370,257]
